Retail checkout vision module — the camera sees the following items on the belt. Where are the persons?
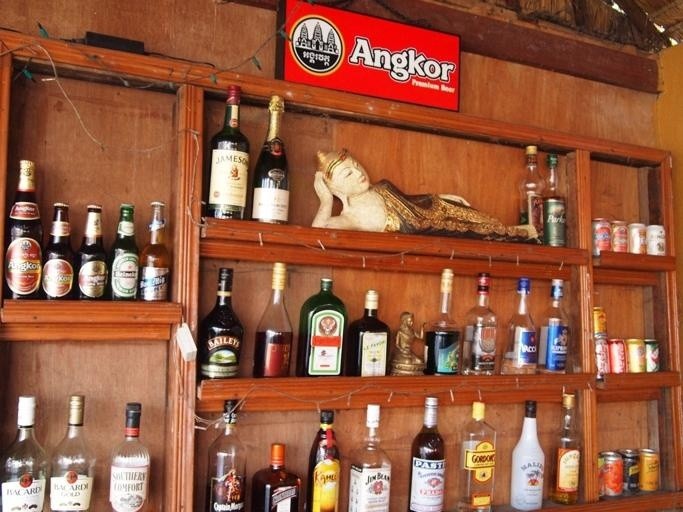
[395,311,427,364]
[310,148,537,243]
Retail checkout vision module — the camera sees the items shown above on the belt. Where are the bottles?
[514,144,546,245]
[4,159,43,301]
[2,394,50,512]
[405,397,447,512]
[509,400,546,511]
[250,441,304,512]
[206,398,249,511]
[297,277,348,376]
[200,266,245,378]
[513,145,567,248]
[500,279,535,373]
[204,85,253,220]
[4,157,171,303]
[49,395,97,512]
[536,279,570,373]
[456,401,497,511]
[546,153,567,246]
[253,93,289,222]
[553,394,583,505]
[109,203,139,302]
[77,203,109,300]
[140,200,171,302]
[419,266,459,377]
[348,403,394,512]
[463,267,496,373]
[105,401,152,511]
[202,84,291,220]
[348,289,391,376]
[44,200,77,300]
[199,261,390,380]
[250,260,295,378]
[0,393,151,511]
[306,409,342,512]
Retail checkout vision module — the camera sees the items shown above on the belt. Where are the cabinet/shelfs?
[0,28,683,512]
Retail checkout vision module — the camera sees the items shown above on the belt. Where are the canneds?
[594,339,611,376]
[645,339,661,373]
[591,218,612,257]
[610,221,629,253]
[627,223,647,255]
[645,225,666,256]
[623,340,647,373]
[592,307,607,338]
[606,339,628,374]
[597,448,659,497]
